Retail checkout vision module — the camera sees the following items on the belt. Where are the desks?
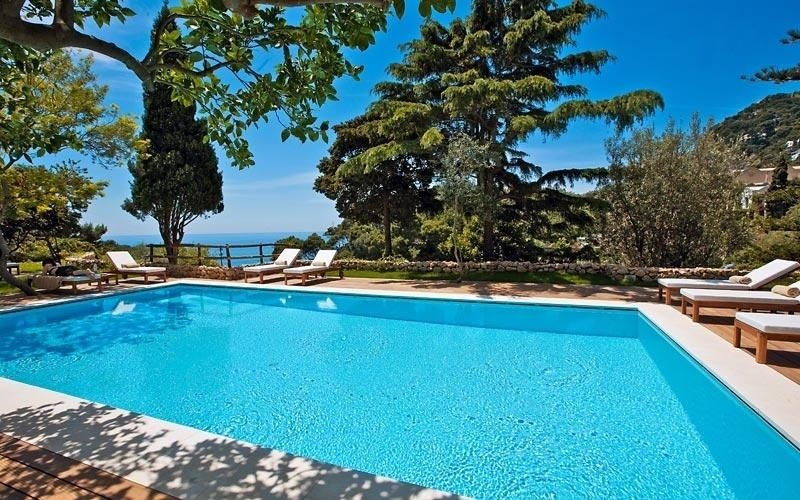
[89,273,118,286]
[6,263,20,275]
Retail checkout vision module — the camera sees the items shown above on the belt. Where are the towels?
[275,261,287,265]
[313,262,325,266]
[121,264,138,268]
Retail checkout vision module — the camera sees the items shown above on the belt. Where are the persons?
[32,258,97,281]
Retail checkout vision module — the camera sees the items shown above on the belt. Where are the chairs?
[283,250,344,285]
[28,274,102,295]
[658,259,800,363]
[242,247,300,284]
[105,250,167,284]
